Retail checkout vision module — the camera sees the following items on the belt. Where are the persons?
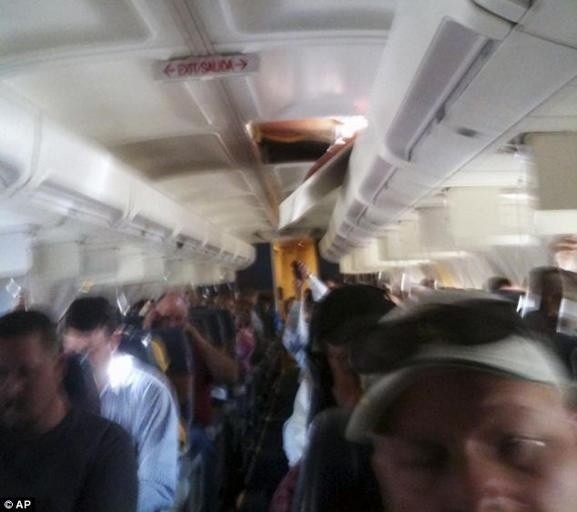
[0,259,577,512]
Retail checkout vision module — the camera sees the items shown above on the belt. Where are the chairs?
[119,328,157,365]
[222,307,235,355]
[193,308,214,350]
[63,352,95,411]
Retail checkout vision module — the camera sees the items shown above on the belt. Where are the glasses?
[349,305,520,375]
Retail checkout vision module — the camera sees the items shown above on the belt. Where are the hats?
[310,288,391,345]
[345,288,560,442]
